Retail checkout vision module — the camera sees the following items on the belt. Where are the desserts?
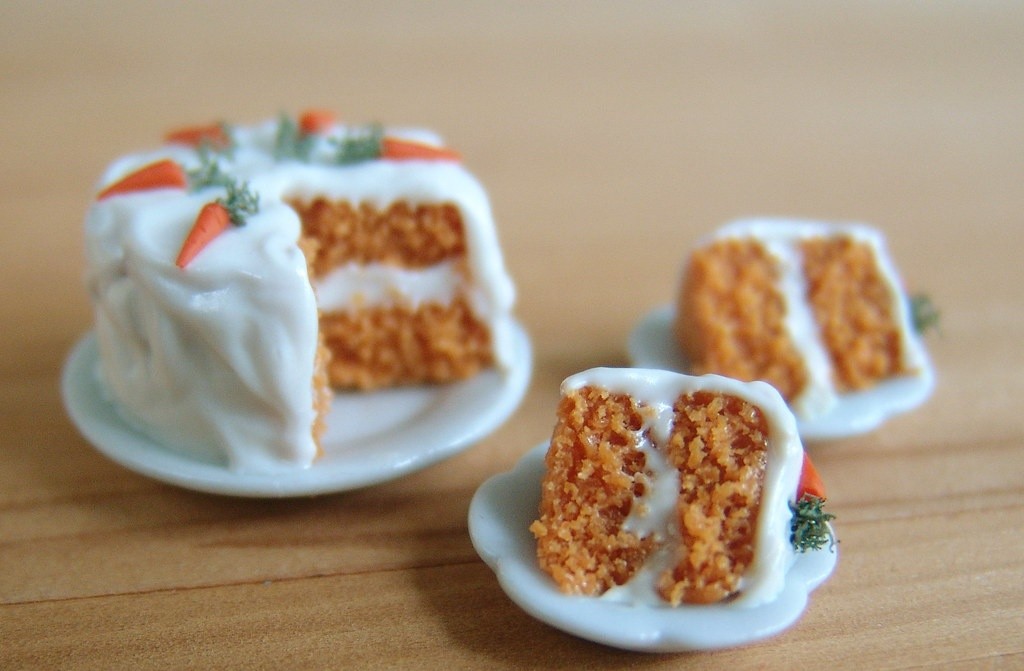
[529,364,837,612]
[80,111,525,479]
[676,218,929,419]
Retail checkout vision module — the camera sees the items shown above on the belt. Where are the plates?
[468,435,839,653]
[58,317,533,500]
[623,301,937,440]
